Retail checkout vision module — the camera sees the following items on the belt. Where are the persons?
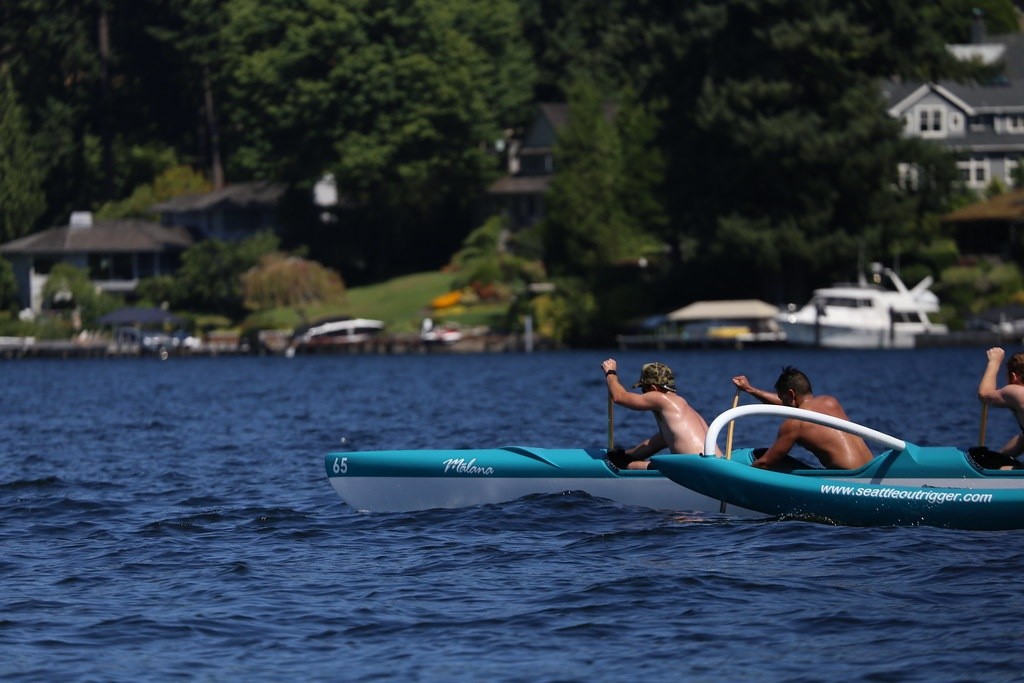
[601,358,724,470]
[970,346,1024,470]
[285,308,313,357]
[732,367,873,471]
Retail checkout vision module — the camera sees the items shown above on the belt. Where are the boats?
[776,288,947,350]
[294,316,384,345]
[648,403,1024,532]
[326,445,769,519]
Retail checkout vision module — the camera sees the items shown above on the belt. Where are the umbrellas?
[97,303,186,343]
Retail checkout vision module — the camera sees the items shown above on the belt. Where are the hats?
[630,361,675,389]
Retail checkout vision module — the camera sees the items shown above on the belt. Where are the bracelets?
[606,370,616,377]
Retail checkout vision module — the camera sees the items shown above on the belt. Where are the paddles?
[607,393,614,449]
[981,404,986,450]
[718,386,741,513]
